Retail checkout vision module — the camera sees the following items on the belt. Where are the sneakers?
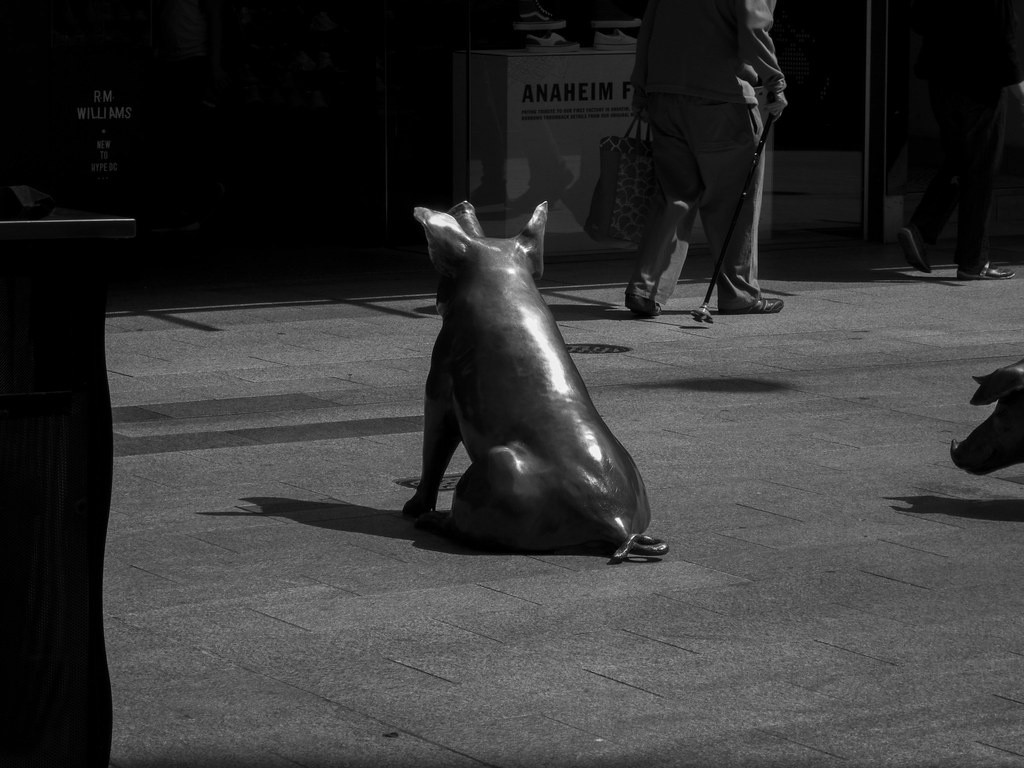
[593,28,638,51]
[524,31,580,51]
[590,6,642,28]
[719,296,784,314]
[897,222,931,273]
[625,293,662,315]
[957,261,1016,280]
[513,1,566,30]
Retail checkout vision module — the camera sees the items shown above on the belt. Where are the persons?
[625,0,787,316]
[899,1,1024,279]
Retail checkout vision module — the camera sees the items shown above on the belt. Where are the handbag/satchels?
[582,111,667,250]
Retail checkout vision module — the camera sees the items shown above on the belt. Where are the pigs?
[400,198,669,563]
[949,359,1024,478]
